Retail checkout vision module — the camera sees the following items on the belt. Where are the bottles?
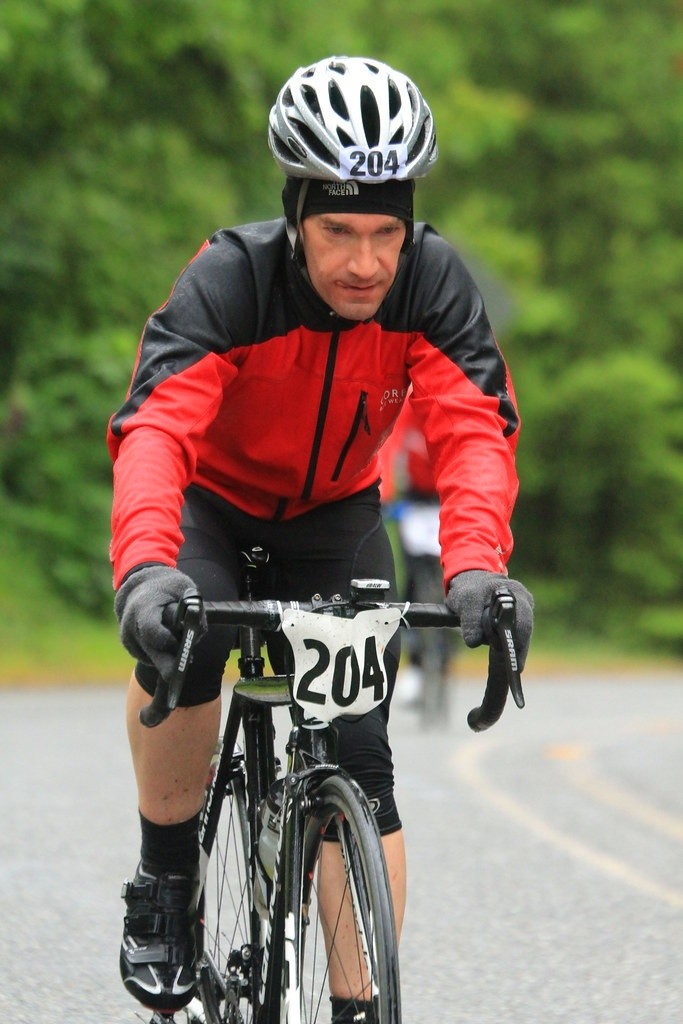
[254,779,298,919]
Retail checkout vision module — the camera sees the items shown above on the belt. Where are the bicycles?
[132,544,527,1024]
[390,504,456,731]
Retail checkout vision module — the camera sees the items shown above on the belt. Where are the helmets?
[268,54,440,184]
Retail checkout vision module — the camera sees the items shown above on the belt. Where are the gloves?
[444,569,535,672]
[113,565,210,682]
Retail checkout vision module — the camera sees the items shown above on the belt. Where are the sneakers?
[119,860,200,1012]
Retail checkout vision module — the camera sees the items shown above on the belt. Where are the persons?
[380,385,461,713]
[105,55,536,1023]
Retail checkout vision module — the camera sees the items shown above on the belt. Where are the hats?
[282,176,414,239]
